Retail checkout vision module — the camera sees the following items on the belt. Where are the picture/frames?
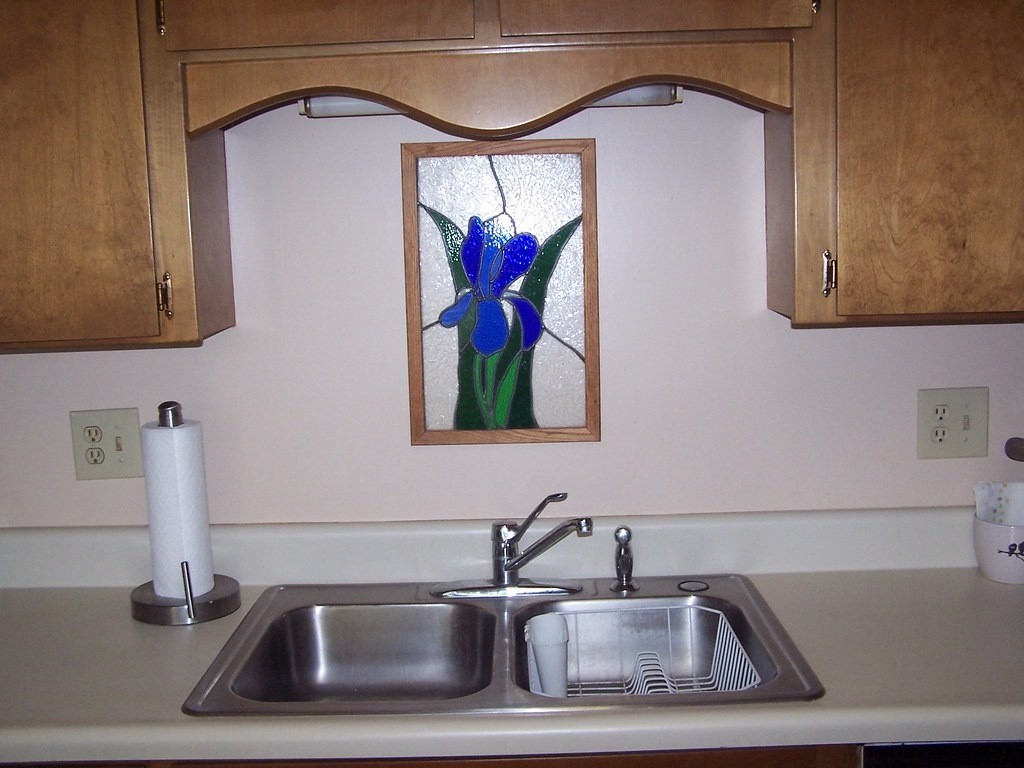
[400,138,601,446]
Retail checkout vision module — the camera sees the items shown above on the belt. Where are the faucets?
[490,492,594,588]
[609,523,640,593]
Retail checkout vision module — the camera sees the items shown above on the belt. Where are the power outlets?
[916,387,989,461]
[70,408,143,481]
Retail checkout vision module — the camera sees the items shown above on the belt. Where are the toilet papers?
[139,416,216,600]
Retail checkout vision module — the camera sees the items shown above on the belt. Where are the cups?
[972,514,1024,586]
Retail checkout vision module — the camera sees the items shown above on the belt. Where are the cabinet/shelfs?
[159,0,816,49]
[0,0,237,352]
[763,0,1024,327]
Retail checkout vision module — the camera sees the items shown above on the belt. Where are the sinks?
[500,572,826,716]
[181,578,513,717]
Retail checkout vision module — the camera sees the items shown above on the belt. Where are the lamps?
[297,84,684,118]
